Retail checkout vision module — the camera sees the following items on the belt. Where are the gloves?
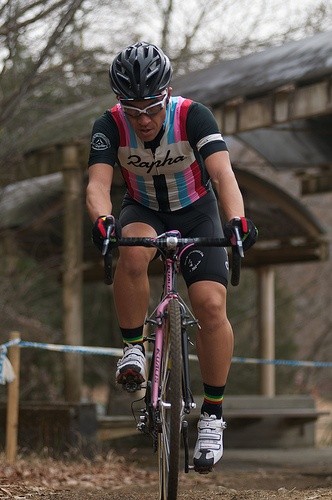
[92,215,123,255]
[225,216,258,250]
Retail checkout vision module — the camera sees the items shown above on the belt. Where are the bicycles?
[101,216,245,500]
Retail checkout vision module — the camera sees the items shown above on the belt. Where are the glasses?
[118,94,168,117]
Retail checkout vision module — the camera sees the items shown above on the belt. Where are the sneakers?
[116,348,146,384]
[193,417,226,470]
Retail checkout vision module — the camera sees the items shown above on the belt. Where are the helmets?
[109,41,173,100]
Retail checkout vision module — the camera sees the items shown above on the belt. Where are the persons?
[86,41,258,475]
